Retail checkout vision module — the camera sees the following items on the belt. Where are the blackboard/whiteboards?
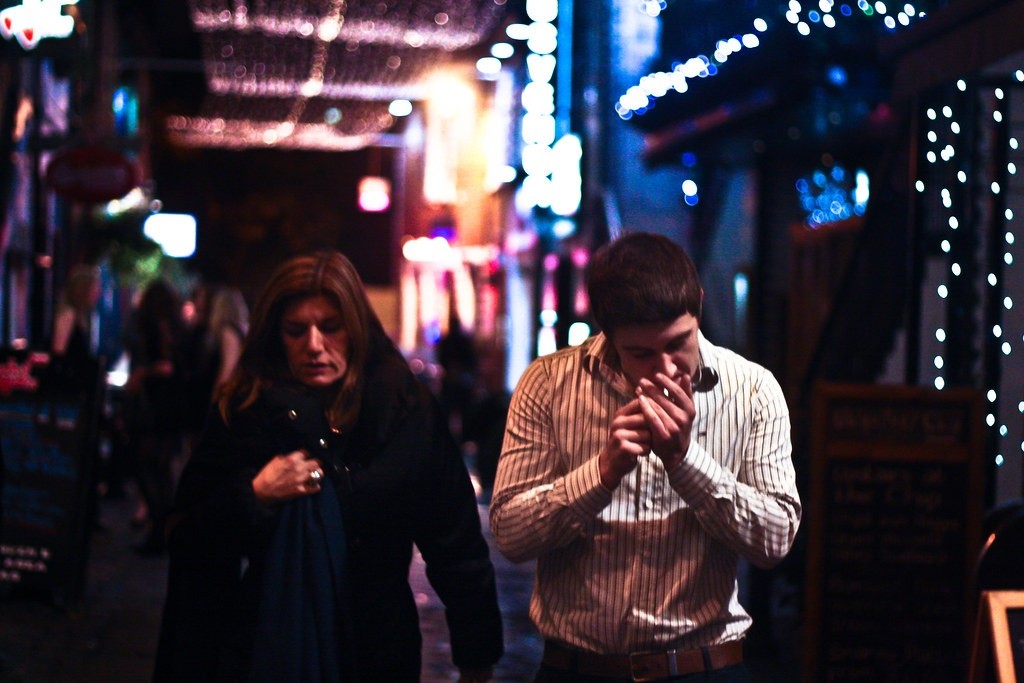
[802,381,983,683]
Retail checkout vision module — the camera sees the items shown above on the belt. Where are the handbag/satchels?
[151,381,346,683]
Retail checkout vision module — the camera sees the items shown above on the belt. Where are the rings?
[310,470,322,486]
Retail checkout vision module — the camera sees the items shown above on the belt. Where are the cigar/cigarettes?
[663,388,669,397]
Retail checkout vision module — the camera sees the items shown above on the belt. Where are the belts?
[541,637,743,683]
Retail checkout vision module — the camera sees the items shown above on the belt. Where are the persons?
[49,262,248,556]
[436,317,484,420]
[489,228,801,683]
[172,248,505,683]
